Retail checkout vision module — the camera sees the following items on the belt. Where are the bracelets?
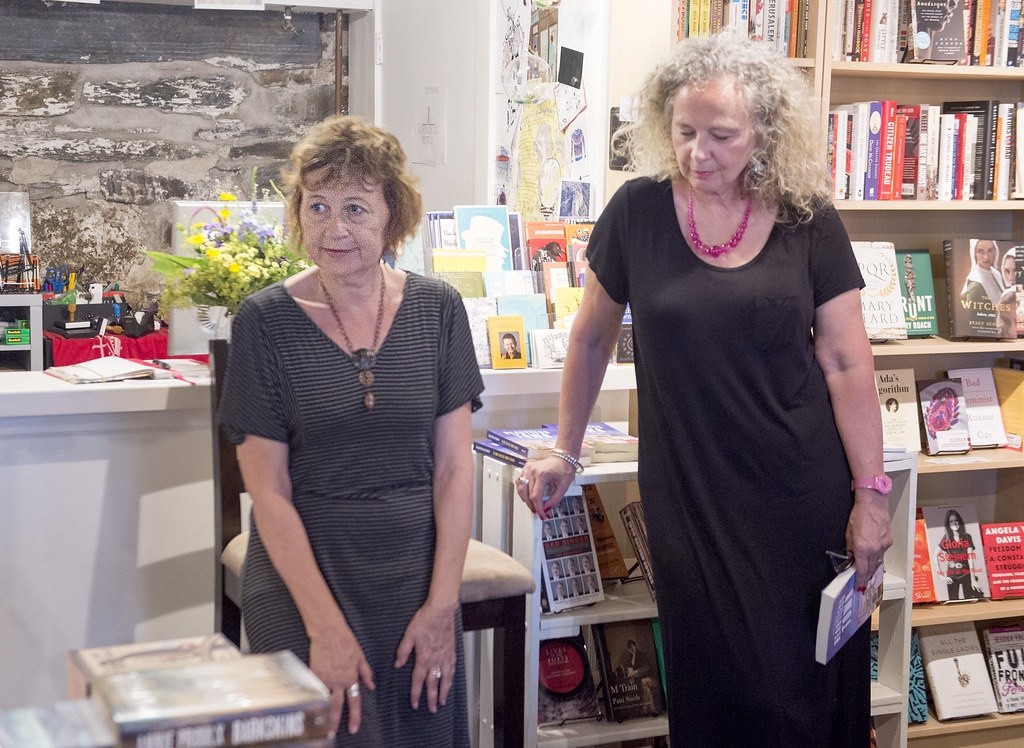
[551,448,584,474]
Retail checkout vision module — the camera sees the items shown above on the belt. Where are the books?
[42,356,211,385]
[538,626,601,728]
[473,422,638,468]
[590,619,667,722]
[815,561,884,663]
[0,633,331,748]
[678,0,1024,725]
[426,205,634,368]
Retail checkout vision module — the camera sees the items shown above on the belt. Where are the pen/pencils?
[154,360,170,370]
[43,282,52,290]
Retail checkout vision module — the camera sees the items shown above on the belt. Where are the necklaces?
[688,185,752,258]
[316,267,386,410]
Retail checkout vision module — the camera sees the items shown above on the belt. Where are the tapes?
[134,312,145,324]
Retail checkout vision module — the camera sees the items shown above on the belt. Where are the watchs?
[852,474,893,495]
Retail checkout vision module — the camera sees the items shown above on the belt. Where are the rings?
[517,477,529,485]
[346,682,360,699]
[430,668,441,678]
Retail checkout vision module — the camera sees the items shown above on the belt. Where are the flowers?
[142,163,311,311]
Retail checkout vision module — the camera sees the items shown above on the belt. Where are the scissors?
[45,266,69,293]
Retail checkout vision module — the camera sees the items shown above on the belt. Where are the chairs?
[209,334,534,747]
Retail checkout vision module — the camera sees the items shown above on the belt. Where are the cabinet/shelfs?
[0,291,43,373]
[471,0,1024,748]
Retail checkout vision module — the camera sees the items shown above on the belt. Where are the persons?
[516,29,893,748]
[933,510,982,600]
[540,495,600,602]
[214,115,485,748]
[501,333,521,359]
[996,248,1017,337]
[626,640,660,717]
[886,398,898,412]
[960,238,1017,338]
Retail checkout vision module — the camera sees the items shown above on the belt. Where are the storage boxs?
[66,636,333,747]
